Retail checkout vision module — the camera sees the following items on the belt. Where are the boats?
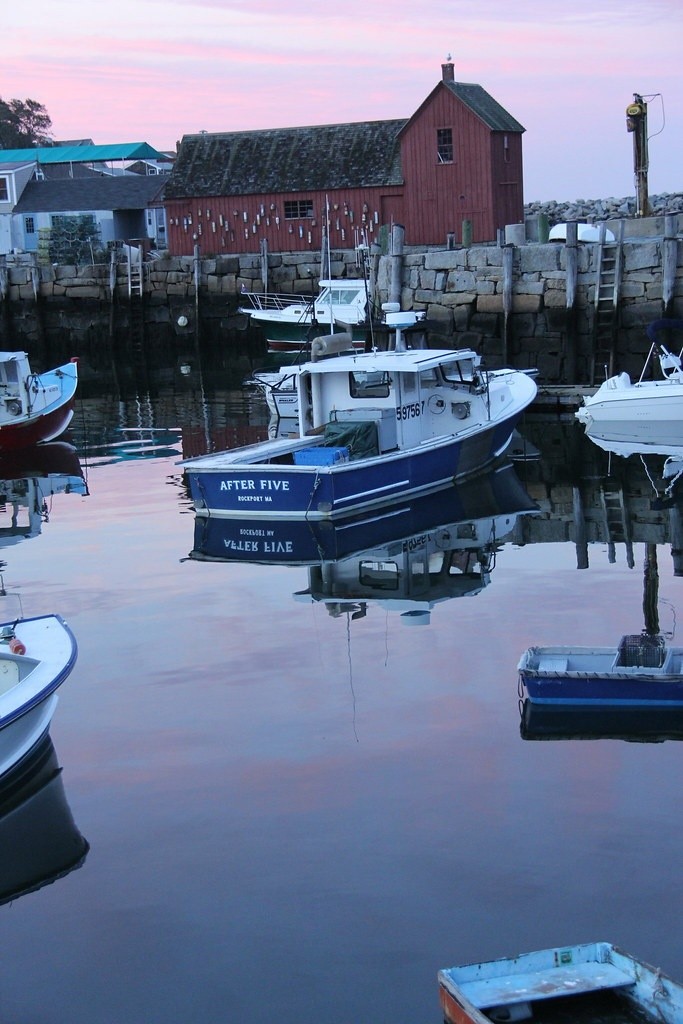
[518,644,683,708]
[437,940,683,1024]
[519,702,683,745]
[549,224,615,244]
[188,464,537,628]
[576,421,683,491]
[0,430,89,543]
[237,279,422,342]
[176,344,537,521]
[0,611,77,775]
[575,340,683,420]
[0,736,93,905]
[1,352,80,446]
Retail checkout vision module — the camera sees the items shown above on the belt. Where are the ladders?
[128,245,143,297]
[591,314,615,384]
[592,218,625,311]
